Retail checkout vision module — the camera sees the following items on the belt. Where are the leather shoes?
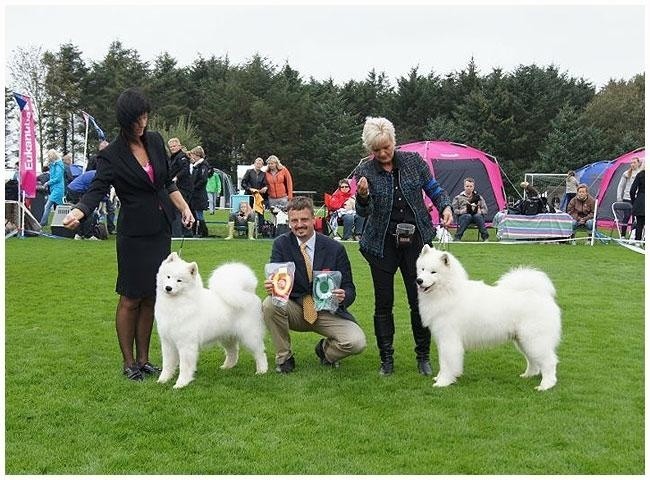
[122,362,144,382]
[137,361,162,375]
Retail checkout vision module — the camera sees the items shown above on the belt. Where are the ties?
[299,242,319,325]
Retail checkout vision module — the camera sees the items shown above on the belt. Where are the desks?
[495,212,575,243]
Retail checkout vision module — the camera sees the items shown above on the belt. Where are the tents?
[348,140,508,231]
[596,145,644,232]
[559,159,616,215]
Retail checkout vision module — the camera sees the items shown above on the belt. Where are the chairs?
[455,215,485,242]
[325,206,356,242]
[607,202,636,240]
[230,195,258,239]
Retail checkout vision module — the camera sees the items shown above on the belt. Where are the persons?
[567,183,596,246]
[328,179,365,241]
[6,141,116,241]
[616,156,643,239]
[224,155,292,240]
[262,196,367,373]
[565,170,577,213]
[453,177,489,242]
[520,181,540,198]
[60,88,196,380]
[165,138,221,237]
[552,194,560,213]
[630,170,645,247]
[356,116,452,377]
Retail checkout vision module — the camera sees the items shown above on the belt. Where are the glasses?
[341,186,349,188]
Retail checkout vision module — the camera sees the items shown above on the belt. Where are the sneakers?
[586,241,591,246]
[314,337,341,369]
[275,355,295,375]
[570,241,576,246]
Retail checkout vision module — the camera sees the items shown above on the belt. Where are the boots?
[373,312,395,376]
[409,310,434,378]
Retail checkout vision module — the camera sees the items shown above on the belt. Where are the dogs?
[153,251,269,390]
[415,243,563,393]
